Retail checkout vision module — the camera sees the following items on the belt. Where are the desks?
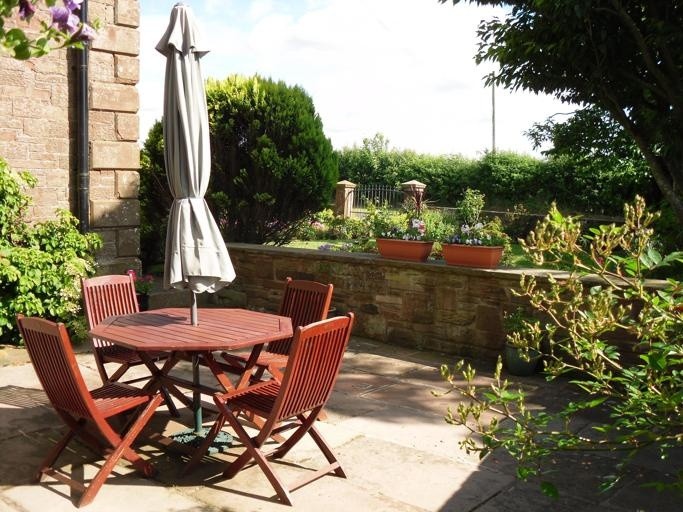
[88,308,293,479]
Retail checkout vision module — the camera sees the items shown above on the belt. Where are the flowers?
[440,215,512,267]
[360,193,444,240]
[128,270,155,295]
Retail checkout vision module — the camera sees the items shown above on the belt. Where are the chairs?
[221,277,334,420]
[213,311,355,506]
[80,274,180,425]
[16,313,165,508]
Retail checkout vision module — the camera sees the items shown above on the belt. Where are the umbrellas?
[154,1,237,433]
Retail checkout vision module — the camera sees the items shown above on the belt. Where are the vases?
[376,238,433,262]
[441,243,504,269]
[137,295,149,311]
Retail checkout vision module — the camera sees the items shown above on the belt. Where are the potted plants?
[502,307,544,376]
